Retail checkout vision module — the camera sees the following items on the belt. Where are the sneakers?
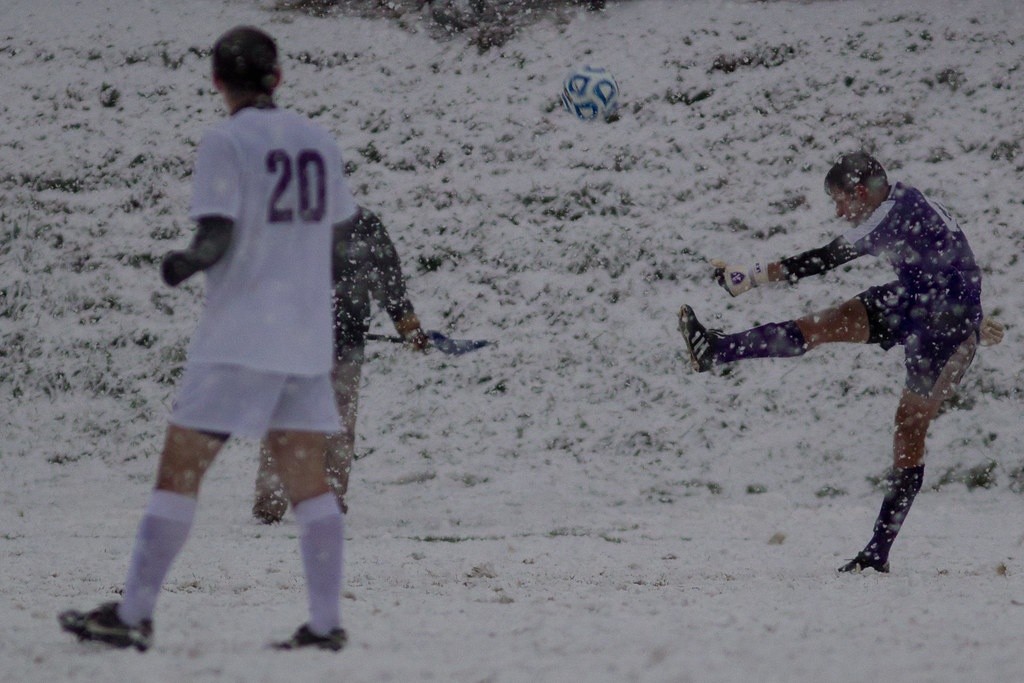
[676,304,724,372]
[838,553,890,575]
[58,602,151,652]
[269,623,346,653]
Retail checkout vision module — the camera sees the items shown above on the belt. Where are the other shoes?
[253,497,288,525]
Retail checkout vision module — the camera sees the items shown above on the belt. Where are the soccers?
[557,61,621,122]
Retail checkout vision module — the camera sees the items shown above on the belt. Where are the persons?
[57,25,359,653]
[251,203,428,524]
[678,153,1007,574]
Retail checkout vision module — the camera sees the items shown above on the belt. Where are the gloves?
[710,256,769,299]
[978,316,1004,346]
